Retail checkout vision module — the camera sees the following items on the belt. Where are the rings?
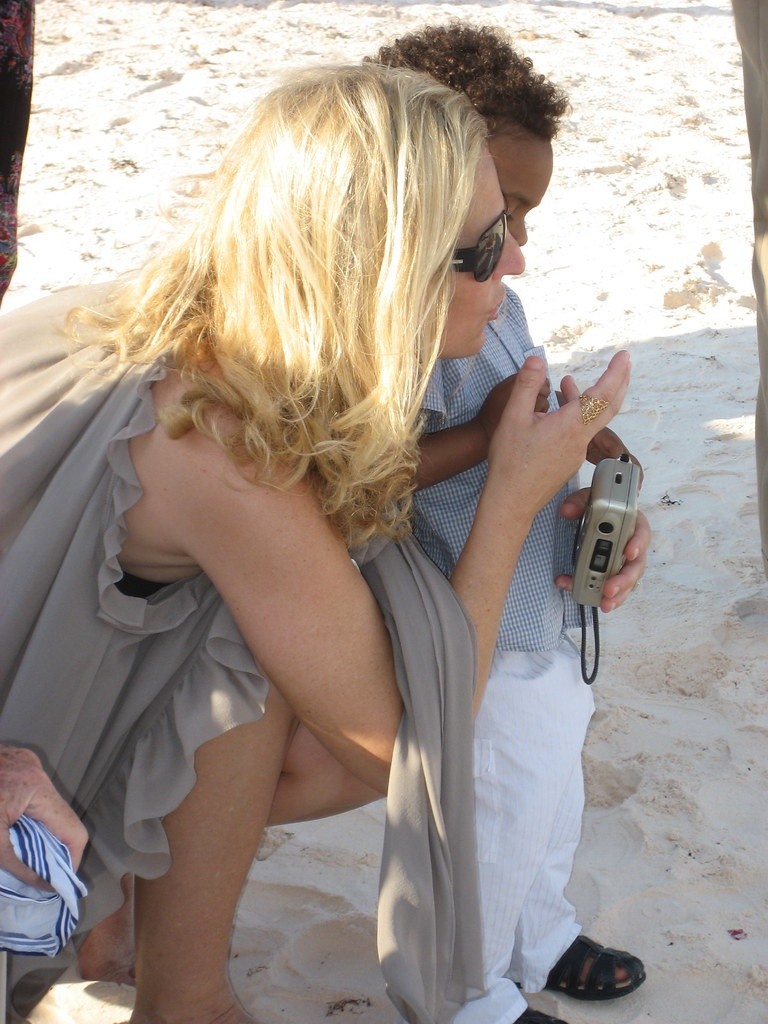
[578,393,610,424]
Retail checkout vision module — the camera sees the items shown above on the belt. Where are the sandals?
[514,1004,570,1024]
[515,933,646,1001]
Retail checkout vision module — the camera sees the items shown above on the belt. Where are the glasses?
[452,199,509,284]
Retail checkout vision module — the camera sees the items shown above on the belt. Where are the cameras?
[572,459,641,608]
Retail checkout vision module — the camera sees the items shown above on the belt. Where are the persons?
[0,0,768,1024]
